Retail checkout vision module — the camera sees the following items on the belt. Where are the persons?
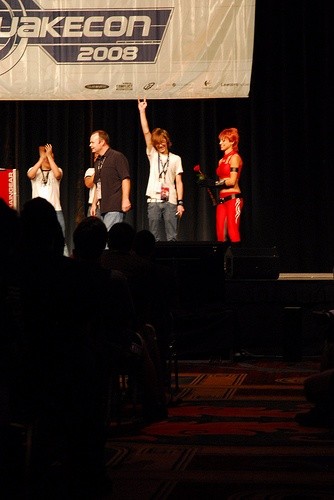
[0,197,167,419]
[137,95,185,240]
[27,144,70,257]
[200,127,244,243]
[89,130,131,249]
[84,152,103,220]
[295,309,334,422]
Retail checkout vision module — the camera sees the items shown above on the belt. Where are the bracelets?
[177,199,183,205]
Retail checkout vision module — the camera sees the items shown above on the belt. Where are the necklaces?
[39,166,49,184]
[158,152,168,168]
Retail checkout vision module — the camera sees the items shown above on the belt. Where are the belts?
[217,193,241,204]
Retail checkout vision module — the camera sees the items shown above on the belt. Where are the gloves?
[199,176,216,188]
